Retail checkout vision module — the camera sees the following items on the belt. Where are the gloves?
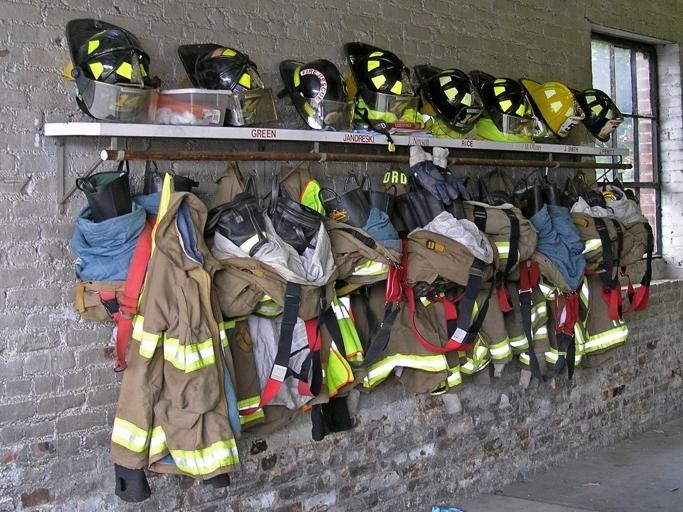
[431,147,471,201]
[411,151,458,205]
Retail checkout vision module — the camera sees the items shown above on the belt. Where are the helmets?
[66,19,162,123]
[471,70,536,143]
[280,59,349,132]
[344,42,419,128]
[519,77,585,140]
[178,43,279,126]
[568,86,623,142]
[415,64,484,140]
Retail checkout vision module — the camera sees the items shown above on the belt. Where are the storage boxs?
[153,86,234,128]
